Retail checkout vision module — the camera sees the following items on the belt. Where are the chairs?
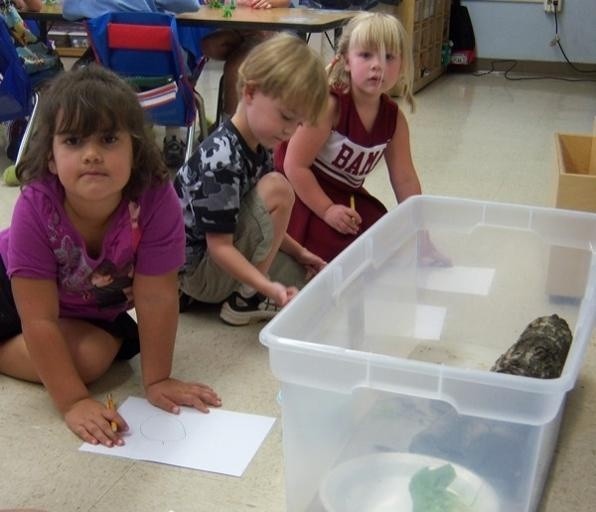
[0,13,59,166]
[82,11,209,166]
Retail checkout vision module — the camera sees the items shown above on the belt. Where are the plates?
[320,451,502,512]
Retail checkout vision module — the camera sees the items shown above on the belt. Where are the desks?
[21,0,362,71]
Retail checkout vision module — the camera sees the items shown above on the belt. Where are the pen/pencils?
[105,390,119,436]
[349,196,355,227]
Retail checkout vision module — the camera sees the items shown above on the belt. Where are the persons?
[273,10,452,269]
[0,63,221,446]
[62,0,207,166]
[1,0,64,162]
[174,34,328,326]
[210,0,308,131]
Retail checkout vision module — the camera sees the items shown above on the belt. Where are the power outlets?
[542,0,563,14]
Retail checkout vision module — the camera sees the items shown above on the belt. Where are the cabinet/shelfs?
[385,1,453,99]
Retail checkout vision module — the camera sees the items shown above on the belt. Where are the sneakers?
[220,289,282,326]
[161,135,187,169]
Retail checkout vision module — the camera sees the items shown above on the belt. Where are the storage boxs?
[257,194,595,511]
